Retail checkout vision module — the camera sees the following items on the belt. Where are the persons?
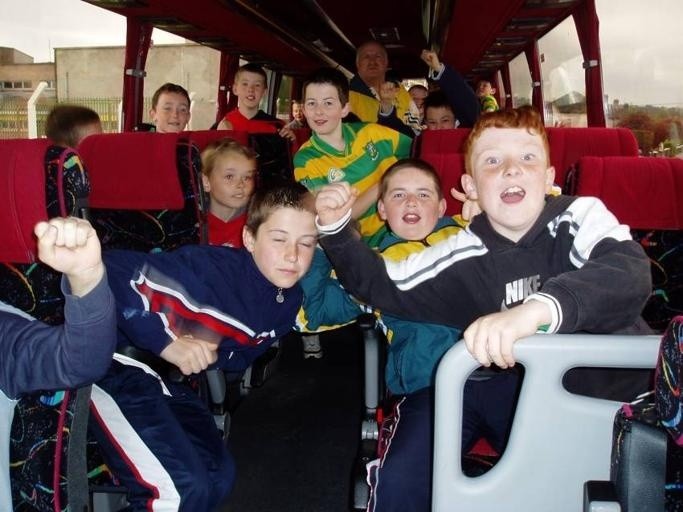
[43,105,102,149]
[315,105,656,512]
[0,214,118,512]
[216,64,297,141]
[286,39,503,141]
[200,138,258,250]
[294,158,525,512]
[89,180,317,512]
[149,82,190,133]
[292,68,414,250]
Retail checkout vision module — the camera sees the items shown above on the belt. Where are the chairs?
[418,127,475,220]
[545,127,639,194]
[409,135,421,161]
[0,136,96,511]
[561,153,683,309]
[176,127,294,397]
[73,132,232,449]
[289,126,312,166]
